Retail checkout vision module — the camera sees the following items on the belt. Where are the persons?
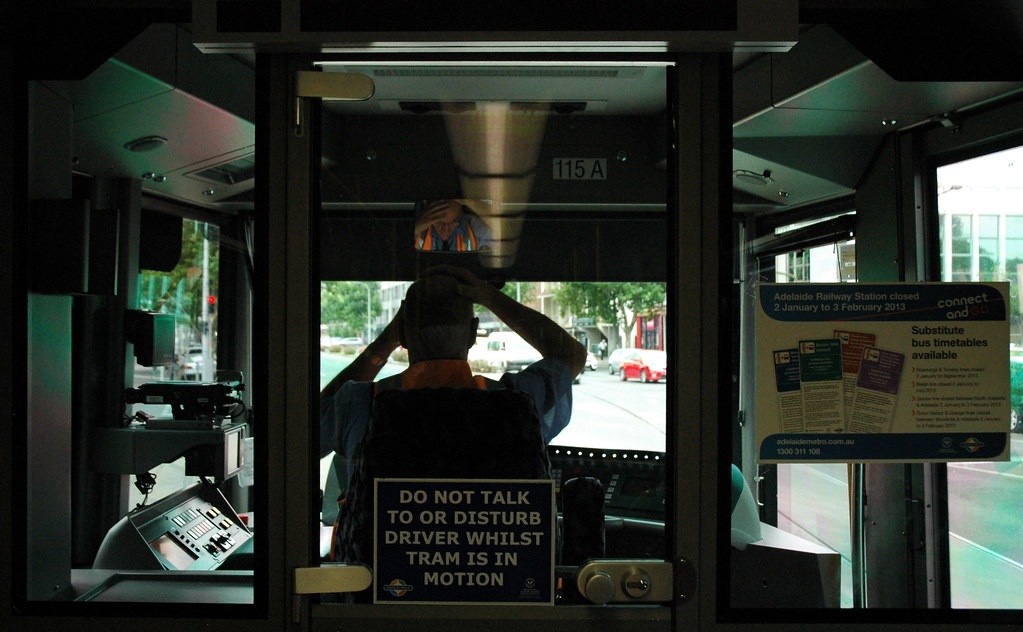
[598,339,607,360]
[414,199,497,251]
[319,265,587,602]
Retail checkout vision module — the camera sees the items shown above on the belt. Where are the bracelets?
[363,350,387,369]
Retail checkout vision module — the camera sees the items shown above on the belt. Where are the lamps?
[441,92,552,275]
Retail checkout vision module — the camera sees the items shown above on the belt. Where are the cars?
[586,351,598,372]
[608,348,666,383]
[1008,356,1023,434]
[181,347,200,380]
[320,335,408,362]
[466,331,544,373]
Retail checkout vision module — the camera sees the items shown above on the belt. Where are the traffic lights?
[208,296,215,313]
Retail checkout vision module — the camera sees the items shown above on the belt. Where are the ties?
[443,241,449,252]
